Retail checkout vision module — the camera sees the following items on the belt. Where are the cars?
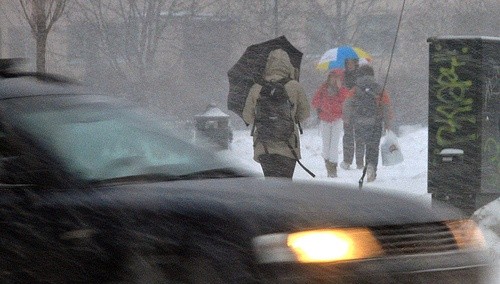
[0,53,498,284]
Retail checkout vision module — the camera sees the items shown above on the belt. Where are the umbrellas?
[226,35,304,129]
[315,46,371,72]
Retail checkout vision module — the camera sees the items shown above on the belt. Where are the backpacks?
[355,82,377,117]
[253,76,295,143]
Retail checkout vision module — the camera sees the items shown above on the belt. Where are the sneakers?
[357,163,364,169]
[367,164,376,182]
[340,161,350,170]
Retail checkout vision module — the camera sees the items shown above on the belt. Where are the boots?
[325,159,337,178]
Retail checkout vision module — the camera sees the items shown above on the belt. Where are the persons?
[312,69,349,177]
[240,49,311,179]
[341,65,392,184]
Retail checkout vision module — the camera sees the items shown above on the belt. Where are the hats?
[361,66,374,77]
